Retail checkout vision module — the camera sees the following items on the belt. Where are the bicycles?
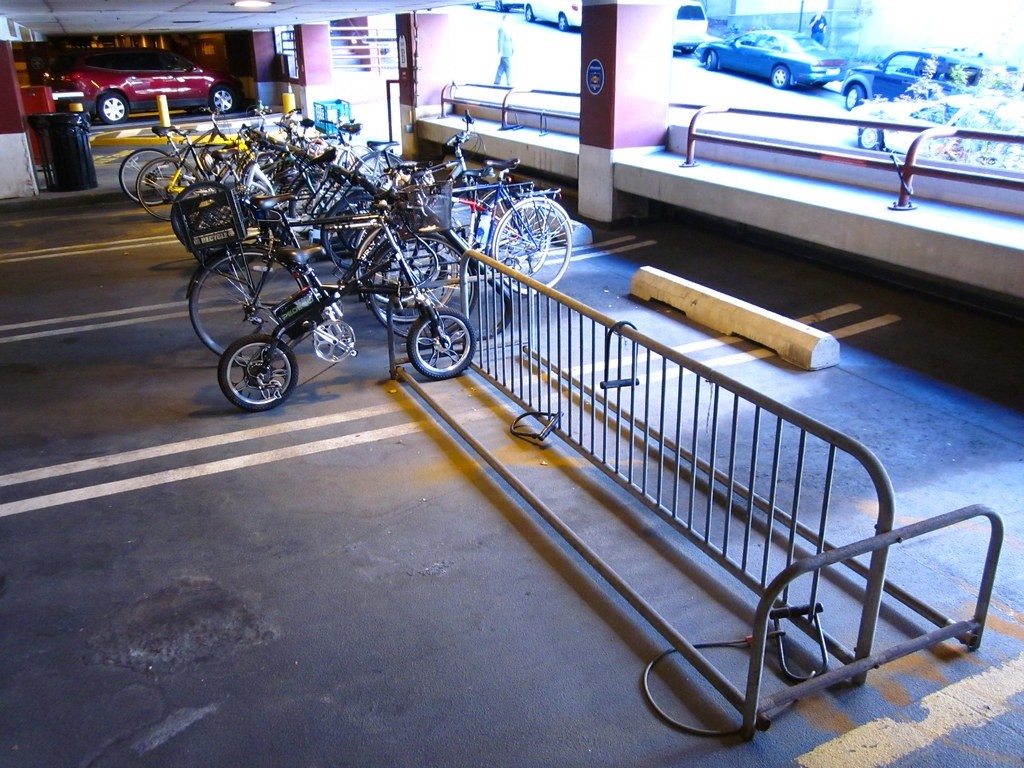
[117,99,576,413]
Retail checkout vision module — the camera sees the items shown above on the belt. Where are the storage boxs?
[177,189,247,253]
[314,100,352,135]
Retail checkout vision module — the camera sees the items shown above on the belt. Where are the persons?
[809,7,827,45]
[493,14,514,86]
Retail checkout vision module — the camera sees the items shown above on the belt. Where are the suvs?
[839,50,1024,112]
[32,46,245,126]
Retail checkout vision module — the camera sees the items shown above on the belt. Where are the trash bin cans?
[27,112,98,192]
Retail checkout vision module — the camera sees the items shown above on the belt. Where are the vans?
[672,0,709,54]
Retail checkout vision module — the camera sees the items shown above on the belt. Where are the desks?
[27,112,98,192]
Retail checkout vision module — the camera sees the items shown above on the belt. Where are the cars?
[472,0,523,13]
[693,29,848,91]
[523,0,583,32]
[855,96,1023,166]
